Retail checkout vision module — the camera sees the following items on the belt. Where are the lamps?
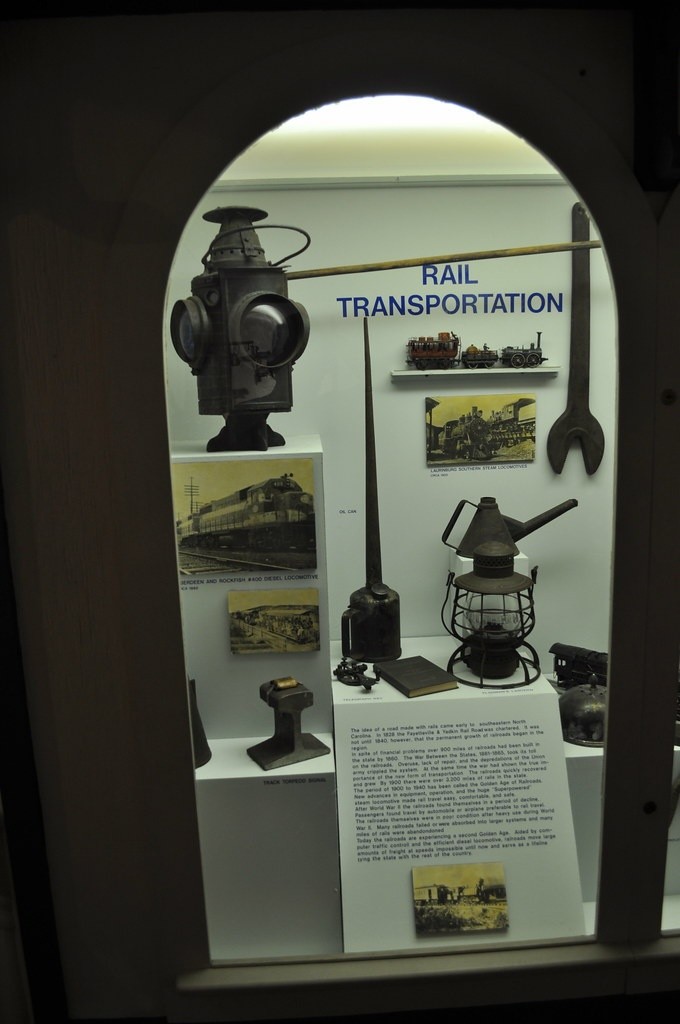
[440,544,544,690]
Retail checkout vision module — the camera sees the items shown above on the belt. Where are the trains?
[405,332,549,369]
[175,474,315,553]
[437,406,500,461]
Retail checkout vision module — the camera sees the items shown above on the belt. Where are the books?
[373,655,459,698]
